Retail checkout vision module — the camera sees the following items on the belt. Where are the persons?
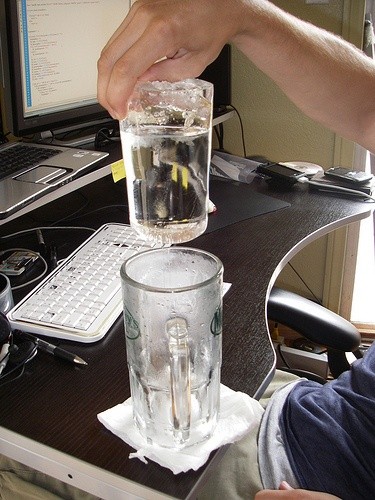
[0,0,375,500]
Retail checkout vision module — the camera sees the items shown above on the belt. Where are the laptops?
[0,114,109,220]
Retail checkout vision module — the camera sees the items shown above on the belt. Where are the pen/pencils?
[14,329,89,365]
[49,241,59,267]
[35,227,51,264]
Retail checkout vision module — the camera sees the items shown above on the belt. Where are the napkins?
[97,381,266,474]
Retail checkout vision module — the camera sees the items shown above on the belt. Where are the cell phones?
[243,155,305,184]
[326,167,374,185]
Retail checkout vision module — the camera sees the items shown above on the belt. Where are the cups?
[119,78,214,245]
[120,247,225,450]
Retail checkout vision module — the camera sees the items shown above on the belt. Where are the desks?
[0,147,375,500]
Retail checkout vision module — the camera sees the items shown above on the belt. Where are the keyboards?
[4,222,173,344]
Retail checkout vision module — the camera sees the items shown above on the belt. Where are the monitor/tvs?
[2,0,167,147]
